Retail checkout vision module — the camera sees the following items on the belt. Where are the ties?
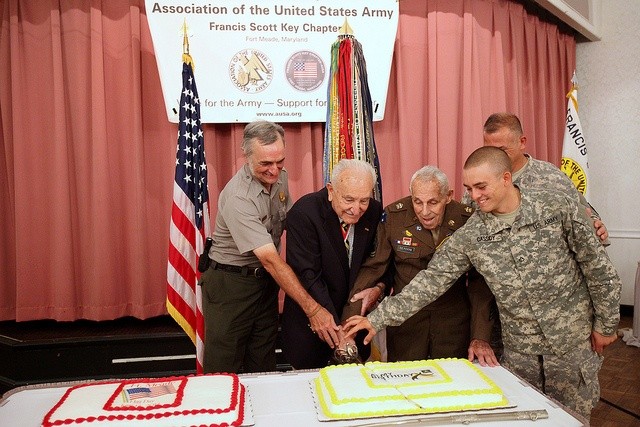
[340,219,351,256]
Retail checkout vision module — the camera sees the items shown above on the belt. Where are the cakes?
[307,357,517,421]
[39,372,246,426]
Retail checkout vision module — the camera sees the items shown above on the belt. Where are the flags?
[164,44,212,375]
[558,85,591,207]
[319,19,397,191]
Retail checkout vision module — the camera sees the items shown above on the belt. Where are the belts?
[208,258,268,277]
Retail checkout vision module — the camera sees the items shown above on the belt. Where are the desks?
[1,358,590,427]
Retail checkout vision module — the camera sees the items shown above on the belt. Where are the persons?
[333,165,501,368]
[306,302,323,320]
[280,158,383,372]
[461,111,613,374]
[342,146,622,426]
[375,284,384,295]
[197,120,341,374]
